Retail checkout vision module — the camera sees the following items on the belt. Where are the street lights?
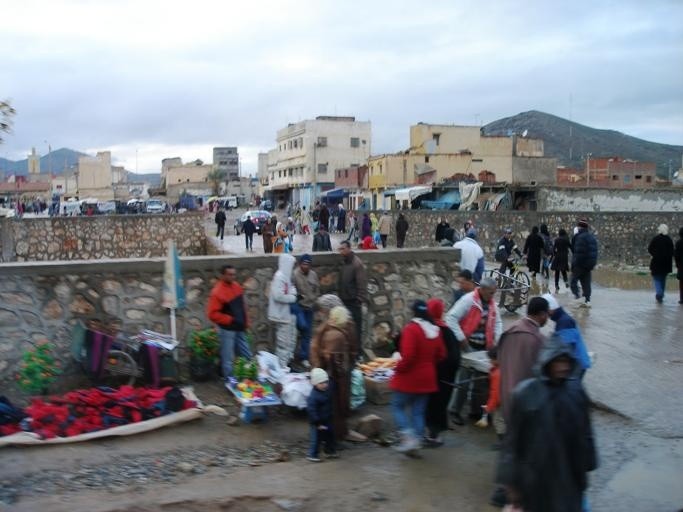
[43,138,52,194]
[604,158,614,186]
[583,150,592,187]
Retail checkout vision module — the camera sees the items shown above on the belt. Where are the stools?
[237,402,269,425]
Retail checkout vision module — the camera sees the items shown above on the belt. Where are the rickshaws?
[474,265,530,311]
[69,321,193,389]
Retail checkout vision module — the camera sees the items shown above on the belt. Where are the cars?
[234,210,273,236]
[0,196,166,218]
[259,200,274,210]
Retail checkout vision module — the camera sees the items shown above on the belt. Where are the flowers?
[15,340,61,393]
[188,327,255,366]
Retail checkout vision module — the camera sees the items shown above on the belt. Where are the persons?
[646,223,673,303]
[207,198,407,254]
[288,254,321,371]
[443,269,502,425]
[336,241,368,353]
[0,184,181,218]
[388,299,460,452]
[672,227,682,304]
[495,337,598,512]
[487,297,549,510]
[543,294,592,385]
[305,295,358,461]
[206,262,254,379]
[433,211,598,309]
[265,252,303,369]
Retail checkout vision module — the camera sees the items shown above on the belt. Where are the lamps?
[519,127,528,138]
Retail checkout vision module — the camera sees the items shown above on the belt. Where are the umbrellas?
[159,240,189,364]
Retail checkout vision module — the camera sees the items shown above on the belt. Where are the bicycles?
[490,254,530,295]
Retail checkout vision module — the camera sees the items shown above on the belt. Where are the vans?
[208,195,238,209]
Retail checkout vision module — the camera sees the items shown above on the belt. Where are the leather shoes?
[452,411,464,425]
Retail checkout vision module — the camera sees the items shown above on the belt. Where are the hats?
[427,300,442,318]
[310,367,330,386]
[317,294,348,327]
